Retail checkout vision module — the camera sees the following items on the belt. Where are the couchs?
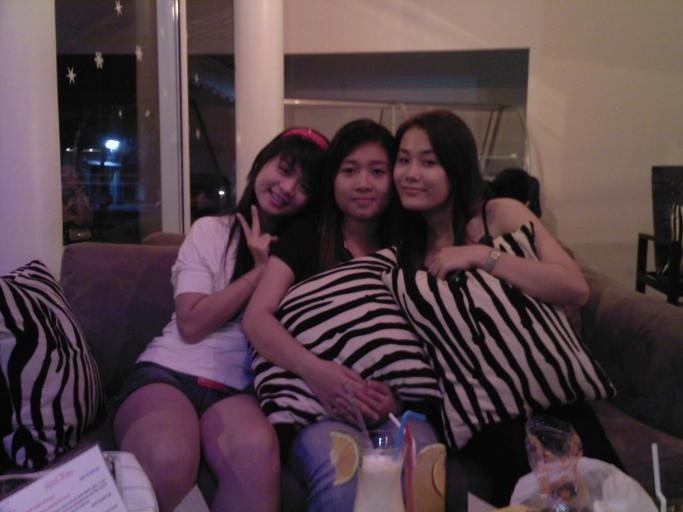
[0,243,683,512]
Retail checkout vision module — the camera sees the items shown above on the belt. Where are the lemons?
[431,450,445,496]
[329,430,360,483]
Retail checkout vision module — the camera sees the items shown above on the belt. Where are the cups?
[352,429,407,511]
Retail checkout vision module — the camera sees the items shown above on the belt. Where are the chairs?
[634,165,683,310]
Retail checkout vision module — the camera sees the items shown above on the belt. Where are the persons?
[111,130,326,512]
[240,119,443,512]
[395,110,627,510]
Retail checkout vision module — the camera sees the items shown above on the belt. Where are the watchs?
[484,245,502,277]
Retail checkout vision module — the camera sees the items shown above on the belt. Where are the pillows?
[1,259,97,472]
